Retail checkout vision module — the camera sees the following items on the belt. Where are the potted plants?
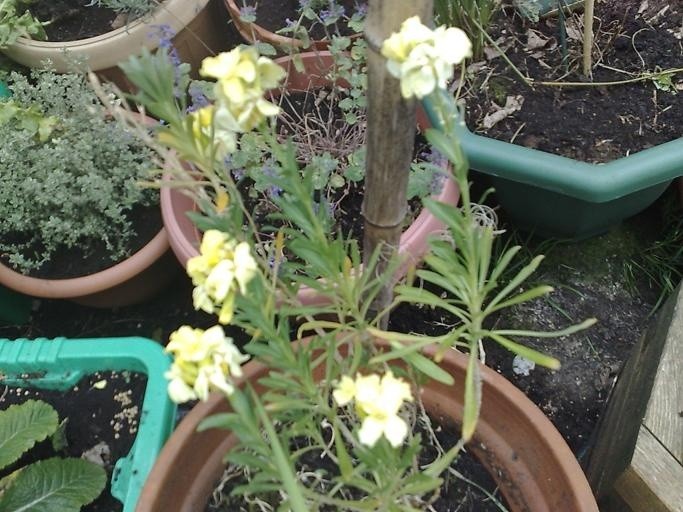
[2,338,178,512]
[226,0,383,55]
[135,332,597,511]
[1,2,211,79]
[2,46,177,309]
[412,4,683,241]
[159,51,464,325]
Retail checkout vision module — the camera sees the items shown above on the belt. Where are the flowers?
[120,13,597,511]
